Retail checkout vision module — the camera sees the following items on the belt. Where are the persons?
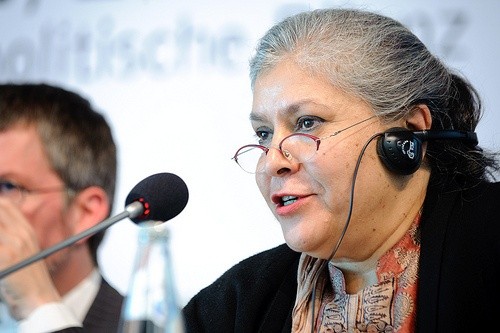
[183,8,500,333]
[0,82,163,333]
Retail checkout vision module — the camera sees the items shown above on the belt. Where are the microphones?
[0,172,189,280]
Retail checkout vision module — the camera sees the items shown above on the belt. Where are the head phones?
[376,128,478,176]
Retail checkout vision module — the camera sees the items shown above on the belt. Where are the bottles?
[119,227,183,333]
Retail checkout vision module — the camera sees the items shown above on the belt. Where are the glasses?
[0,180,65,204]
[231,100,437,174]
